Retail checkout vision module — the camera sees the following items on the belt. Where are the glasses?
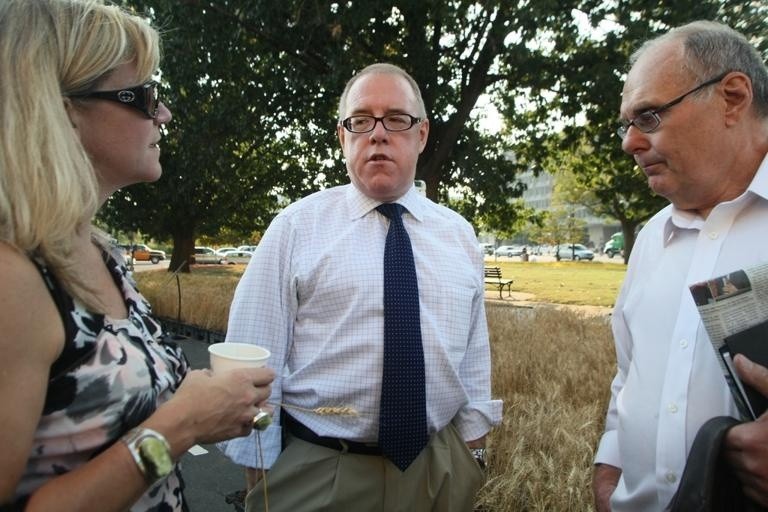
[67,69,165,118]
[617,68,749,141]
[339,115,423,133]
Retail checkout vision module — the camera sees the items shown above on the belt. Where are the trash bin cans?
[522,253,529,263]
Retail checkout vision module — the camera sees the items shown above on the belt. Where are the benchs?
[484,266,515,299]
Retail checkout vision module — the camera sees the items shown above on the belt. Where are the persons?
[0,0,276,512]
[591,20,766,510]
[213,61,507,512]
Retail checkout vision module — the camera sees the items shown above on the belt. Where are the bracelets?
[120,426,177,484]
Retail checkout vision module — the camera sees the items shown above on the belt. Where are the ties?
[374,204,427,474]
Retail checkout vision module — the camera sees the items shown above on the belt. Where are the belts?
[280,408,382,457]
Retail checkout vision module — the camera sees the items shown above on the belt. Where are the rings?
[250,404,272,432]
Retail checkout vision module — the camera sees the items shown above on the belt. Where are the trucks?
[603,230,638,258]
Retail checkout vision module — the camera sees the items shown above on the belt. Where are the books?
[688,265,768,510]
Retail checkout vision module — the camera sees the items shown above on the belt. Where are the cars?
[192,244,258,266]
[479,242,595,262]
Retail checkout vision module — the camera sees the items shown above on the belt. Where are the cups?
[208,342,273,377]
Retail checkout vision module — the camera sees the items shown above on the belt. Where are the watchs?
[467,445,491,469]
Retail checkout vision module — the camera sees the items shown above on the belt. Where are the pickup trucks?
[125,244,166,265]
